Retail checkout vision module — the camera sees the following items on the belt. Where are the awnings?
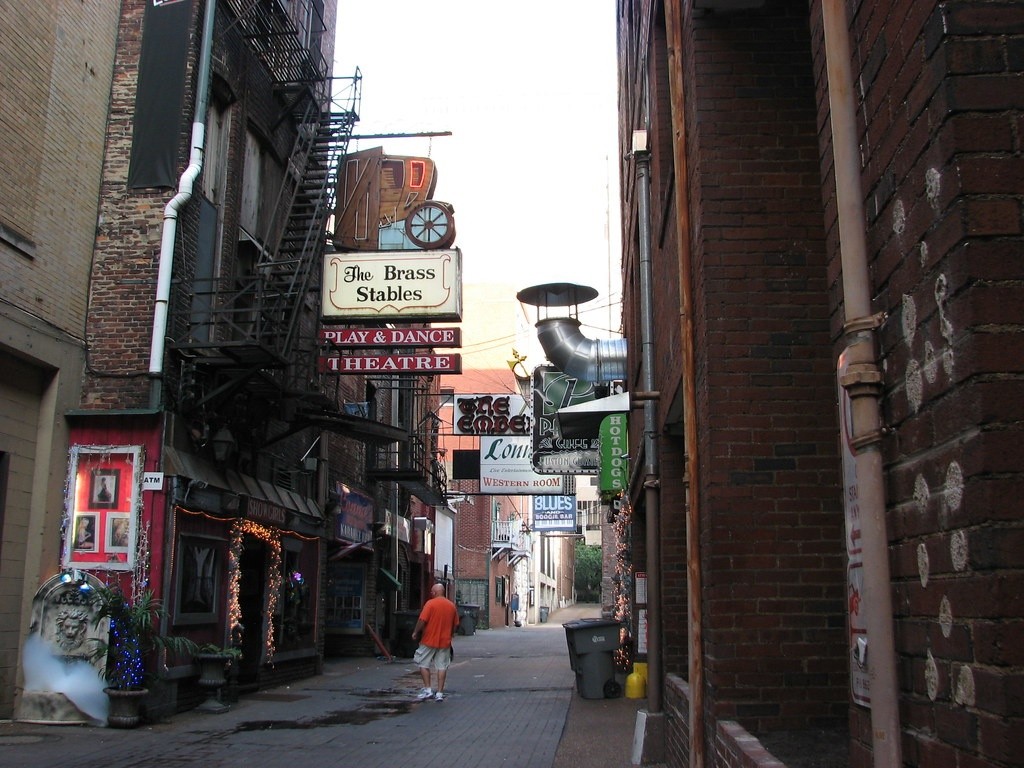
[557,392,630,439]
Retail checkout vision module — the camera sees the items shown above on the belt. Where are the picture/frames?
[61,444,146,572]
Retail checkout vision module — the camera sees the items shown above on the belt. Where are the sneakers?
[417,686,433,699]
[435,692,444,702]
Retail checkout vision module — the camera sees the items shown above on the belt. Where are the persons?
[98,478,111,501]
[77,518,94,547]
[114,519,127,544]
[412,583,459,700]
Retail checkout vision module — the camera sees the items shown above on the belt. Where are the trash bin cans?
[540,606,550,623]
[561,617,624,700]
[392,609,423,660]
[456,603,481,637]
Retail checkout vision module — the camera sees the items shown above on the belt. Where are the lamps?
[183,412,249,462]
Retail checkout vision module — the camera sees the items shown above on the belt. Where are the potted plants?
[81,583,198,726]
[192,644,243,715]
